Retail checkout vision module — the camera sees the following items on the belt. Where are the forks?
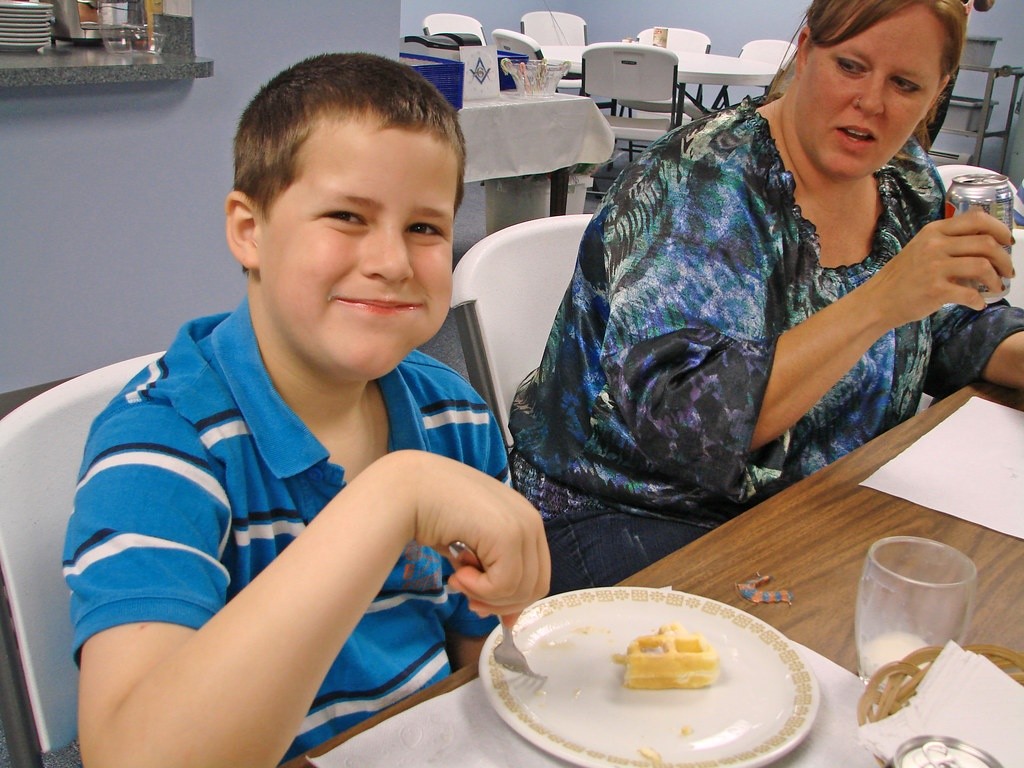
[448,541,548,680]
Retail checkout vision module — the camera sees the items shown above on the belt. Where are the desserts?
[619,623,718,692]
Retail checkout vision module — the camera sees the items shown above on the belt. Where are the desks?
[541,44,782,180]
[275,378,1024,768]
[451,88,615,265]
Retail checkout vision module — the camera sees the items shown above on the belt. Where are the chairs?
[575,42,688,205]
[520,11,617,116]
[710,38,800,117]
[0,348,172,768]
[424,13,488,46]
[492,27,559,93]
[615,27,711,165]
[414,213,595,449]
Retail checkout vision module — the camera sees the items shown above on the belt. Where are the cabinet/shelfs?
[924,65,1024,168]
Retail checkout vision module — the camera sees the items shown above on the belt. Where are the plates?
[0,2,53,51]
[479,587,820,767]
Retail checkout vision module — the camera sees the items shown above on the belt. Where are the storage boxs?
[939,94,1000,132]
[959,36,1004,67]
[497,48,529,90]
[401,52,464,112]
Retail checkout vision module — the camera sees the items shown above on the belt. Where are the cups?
[854,536,976,692]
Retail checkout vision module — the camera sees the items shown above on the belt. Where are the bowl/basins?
[500,58,572,101]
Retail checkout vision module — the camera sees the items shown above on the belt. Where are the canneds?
[653,27,668,49]
[945,172,1013,304]
[882,734,1004,768]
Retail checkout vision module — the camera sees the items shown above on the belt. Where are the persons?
[61,52,551,767]
[509,0,1024,599]
